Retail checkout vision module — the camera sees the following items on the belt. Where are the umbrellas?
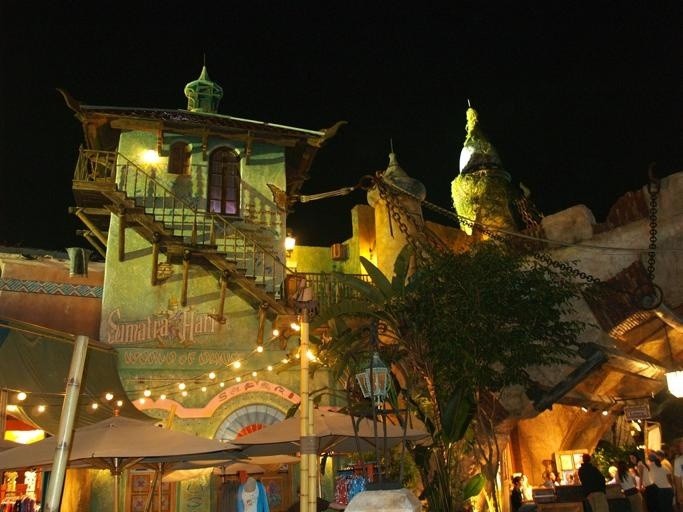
[1,408,243,512]
[230,397,430,500]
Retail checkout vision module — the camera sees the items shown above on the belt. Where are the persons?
[511,476,525,511]
[657,449,673,481]
[628,454,659,512]
[605,464,619,485]
[285,488,347,512]
[648,454,674,512]
[235,475,271,512]
[545,471,560,486]
[614,460,644,512]
[672,452,683,512]
[577,453,610,512]
